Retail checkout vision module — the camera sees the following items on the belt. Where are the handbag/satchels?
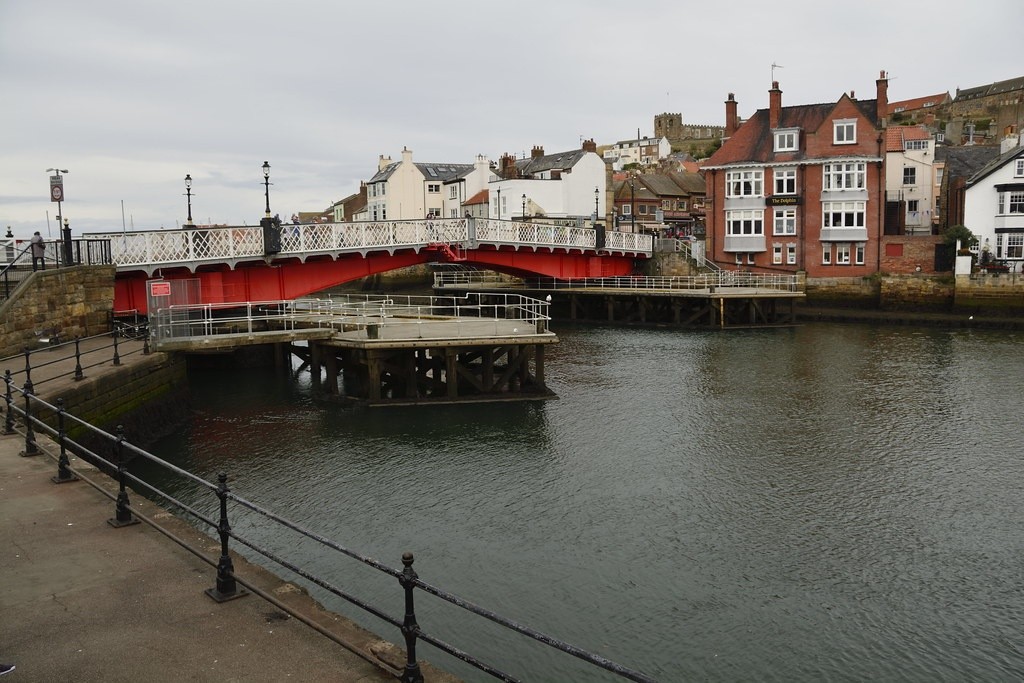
[37,238,45,249]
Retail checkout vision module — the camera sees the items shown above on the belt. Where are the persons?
[465,210,472,218]
[292,215,321,246]
[32,231,46,271]
[649,227,690,238]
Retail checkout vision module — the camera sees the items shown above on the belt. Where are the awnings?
[641,223,670,229]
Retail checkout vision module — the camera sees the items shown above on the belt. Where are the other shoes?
[34,269,37,272]
[42,269,45,270]
[0,663,16,675]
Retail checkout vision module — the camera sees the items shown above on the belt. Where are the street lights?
[46,167,68,267]
[631,168,637,233]
[259,161,274,219]
[594,189,599,220]
[521,194,527,222]
[182,174,195,225]
